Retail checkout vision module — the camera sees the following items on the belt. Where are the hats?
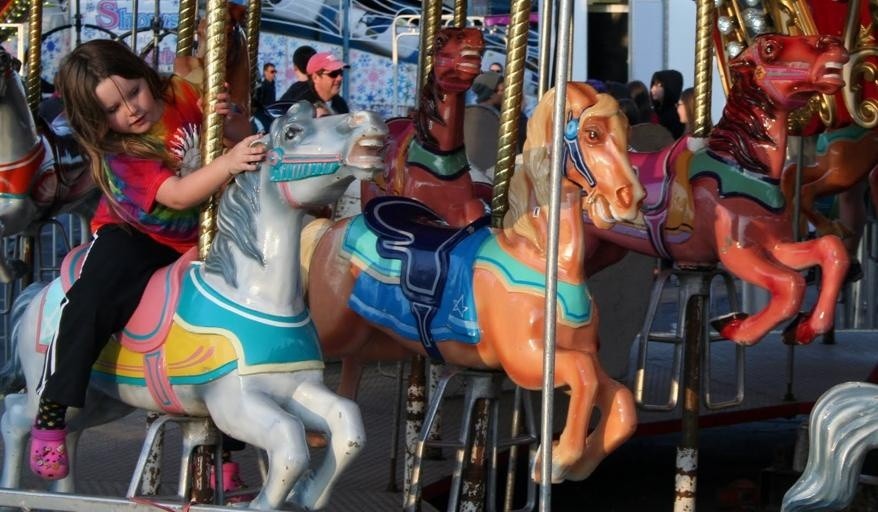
[306,53,350,75]
[472,73,503,102]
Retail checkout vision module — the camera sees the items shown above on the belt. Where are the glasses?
[269,70,276,74]
[321,70,343,78]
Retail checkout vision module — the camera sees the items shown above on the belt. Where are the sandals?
[29,417,69,481]
[209,462,253,502]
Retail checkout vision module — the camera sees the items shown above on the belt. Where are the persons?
[472,63,527,137]
[804,179,868,289]
[255,45,351,132]
[41,73,65,122]
[628,70,694,139]
[31,41,266,510]
